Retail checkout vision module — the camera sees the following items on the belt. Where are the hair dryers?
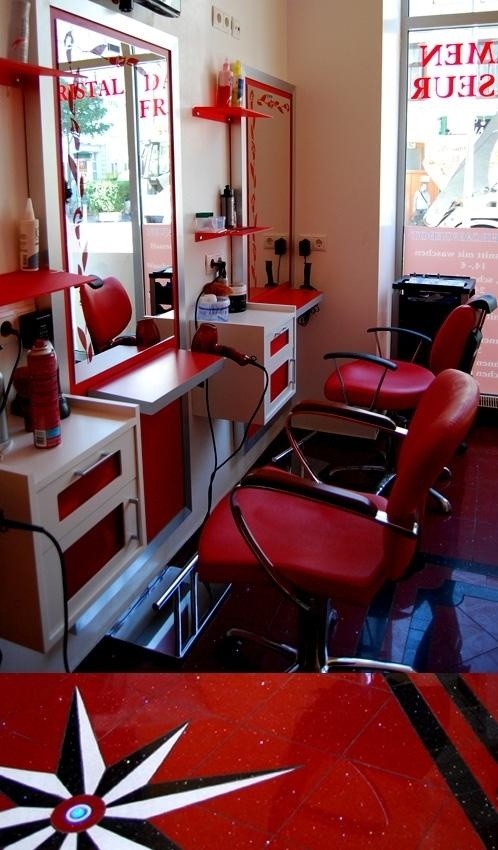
[115,319,160,349]
[192,322,252,387]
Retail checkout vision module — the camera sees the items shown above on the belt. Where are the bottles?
[220,185,235,229]
[217,56,246,107]
[16,196,42,274]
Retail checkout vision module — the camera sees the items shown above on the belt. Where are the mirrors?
[228,62,295,309]
[22,1,187,395]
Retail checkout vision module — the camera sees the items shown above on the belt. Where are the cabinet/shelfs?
[0,56,96,307]
[0,391,147,655]
[390,273,477,457]
[149,264,174,315]
[191,309,297,425]
[143,309,176,343]
[192,104,274,242]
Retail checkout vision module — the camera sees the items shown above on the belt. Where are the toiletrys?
[217,57,234,105]
[232,60,245,107]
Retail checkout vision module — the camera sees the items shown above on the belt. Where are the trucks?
[423,115,498,228]
[141,140,170,223]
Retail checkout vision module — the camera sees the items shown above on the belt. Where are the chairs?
[322,293,497,512]
[80,273,137,355]
[198,367,480,673]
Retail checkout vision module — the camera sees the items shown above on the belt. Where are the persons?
[123,198,131,216]
[411,183,431,226]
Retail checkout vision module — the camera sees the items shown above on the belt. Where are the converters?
[275,238,286,255]
[299,239,310,256]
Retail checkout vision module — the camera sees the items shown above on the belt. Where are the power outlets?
[0,305,36,348]
[263,233,288,250]
[300,234,327,252]
[205,254,225,274]
